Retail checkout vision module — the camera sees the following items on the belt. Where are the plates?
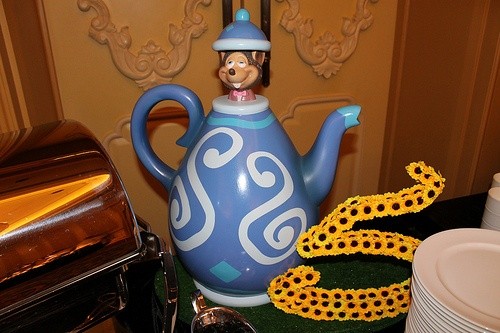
[404,227,499,333]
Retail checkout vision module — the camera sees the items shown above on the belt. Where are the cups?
[190,289,257,333]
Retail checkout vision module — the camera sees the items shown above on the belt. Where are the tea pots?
[129,9,363,308]
